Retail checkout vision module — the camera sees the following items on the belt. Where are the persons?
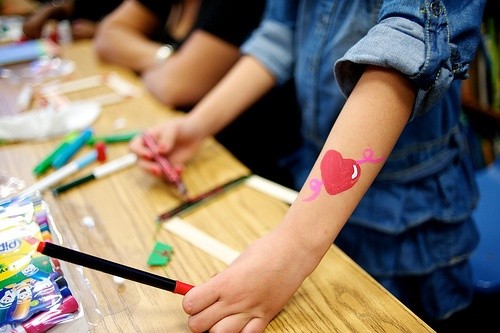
[131,0,482,333]
[0,0,301,186]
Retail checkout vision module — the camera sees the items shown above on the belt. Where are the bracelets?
[153,45,174,66]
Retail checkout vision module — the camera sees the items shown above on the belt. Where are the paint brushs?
[142,130,186,197]
[0,196,78,333]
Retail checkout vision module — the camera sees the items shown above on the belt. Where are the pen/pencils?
[36,240,201,298]
[0,151,138,204]
[158,173,251,222]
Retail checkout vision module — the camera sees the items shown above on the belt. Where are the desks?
[0,39,440,333]
